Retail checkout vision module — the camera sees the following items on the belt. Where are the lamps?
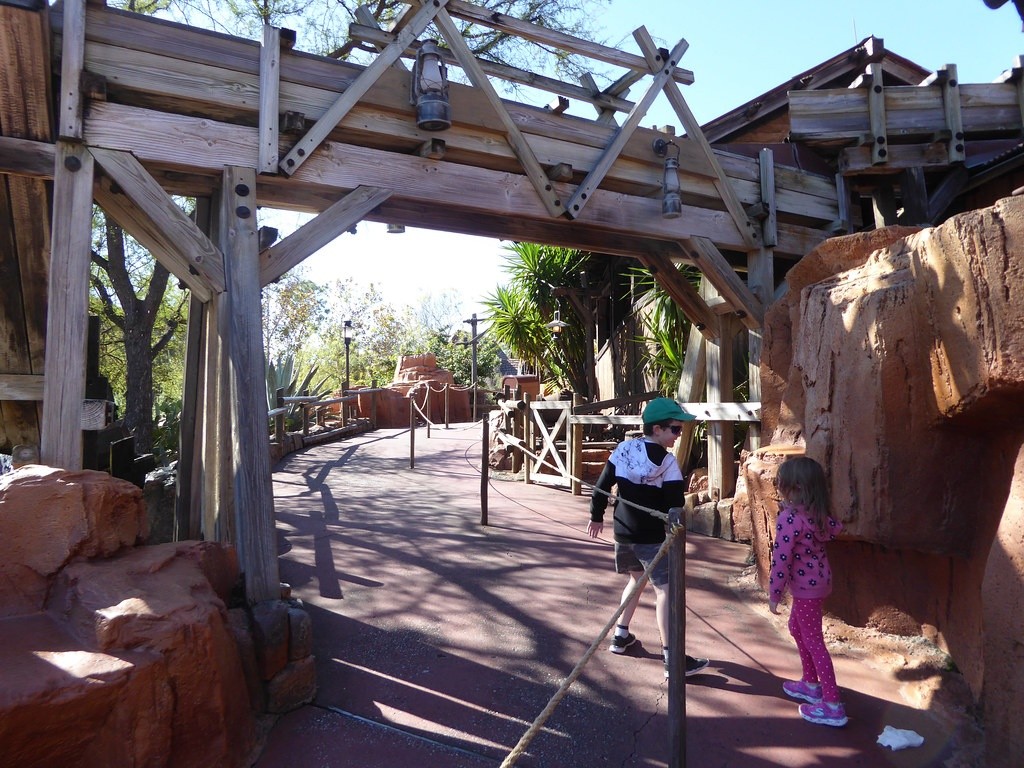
[651,136,686,220]
[408,38,455,133]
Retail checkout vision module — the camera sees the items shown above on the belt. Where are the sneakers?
[664,655,710,678]
[609,633,636,653]
[798,701,848,727]
[783,680,823,704]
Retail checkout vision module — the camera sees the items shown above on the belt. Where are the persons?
[767,456,849,726]
[585,396,709,677]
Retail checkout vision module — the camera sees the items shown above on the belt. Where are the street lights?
[545,286,594,403]
[343,321,353,418]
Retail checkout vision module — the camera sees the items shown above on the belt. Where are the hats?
[642,398,697,424]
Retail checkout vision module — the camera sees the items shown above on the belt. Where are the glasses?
[656,423,682,435]
